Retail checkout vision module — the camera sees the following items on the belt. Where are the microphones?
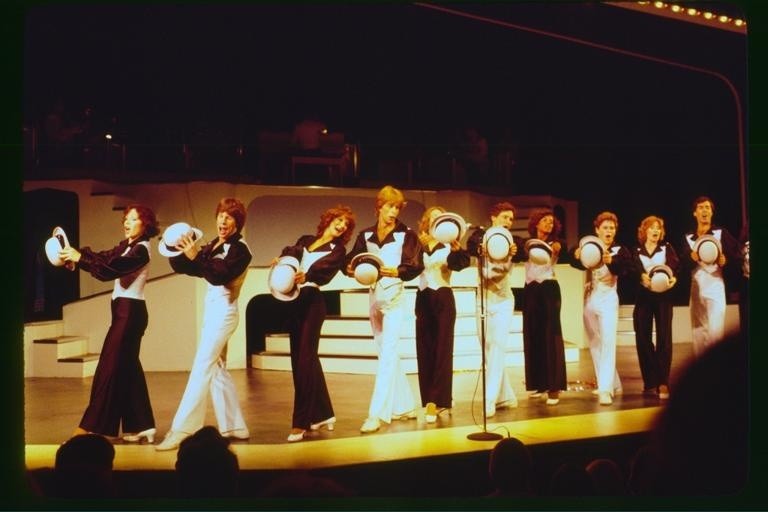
[466,222,482,231]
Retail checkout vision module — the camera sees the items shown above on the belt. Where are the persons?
[464,202,518,418]
[568,212,633,406]
[486,438,538,499]
[678,196,743,355]
[629,215,678,400]
[588,458,623,496]
[288,111,326,159]
[339,186,424,433]
[411,205,470,426]
[43,96,80,165]
[649,334,748,492]
[513,208,567,406]
[468,129,489,177]
[174,427,242,500]
[244,203,355,443]
[153,196,251,451]
[50,435,115,499]
[59,204,161,447]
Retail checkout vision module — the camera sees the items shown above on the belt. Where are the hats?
[158,222,204,258]
[351,252,384,285]
[647,264,673,293]
[693,235,723,274]
[267,255,300,302]
[429,215,607,270]
[45,226,75,268]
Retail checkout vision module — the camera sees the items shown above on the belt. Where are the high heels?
[287,416,336,442]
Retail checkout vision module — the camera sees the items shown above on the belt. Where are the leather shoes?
[530,392,559,405]
[591,389,623,405]
[220,428,249,439]
[482,398,518,418]
[122,428,193,451]
[360,402,452,432]
[659,393,669,399]
